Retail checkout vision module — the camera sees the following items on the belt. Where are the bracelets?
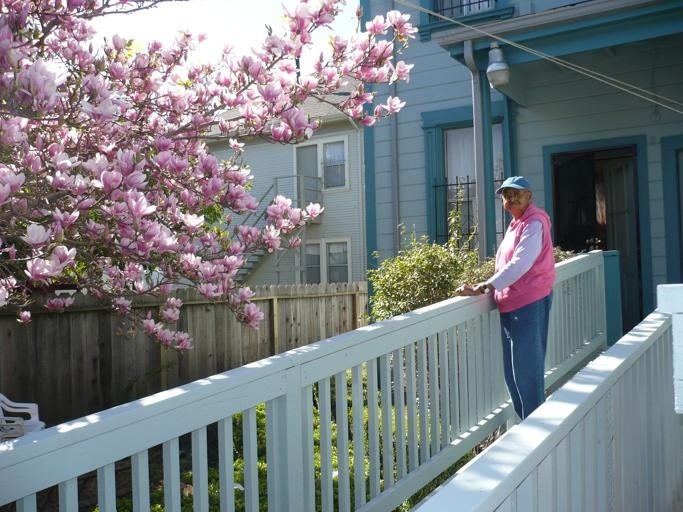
[479,283,490,295]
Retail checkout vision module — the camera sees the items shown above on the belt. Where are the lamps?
[486,44,511,90]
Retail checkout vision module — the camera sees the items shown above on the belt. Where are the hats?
[496,176,529,194]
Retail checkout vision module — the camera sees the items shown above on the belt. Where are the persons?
[453,175,556,420]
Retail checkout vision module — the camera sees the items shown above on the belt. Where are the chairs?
[0,392,46,444]
